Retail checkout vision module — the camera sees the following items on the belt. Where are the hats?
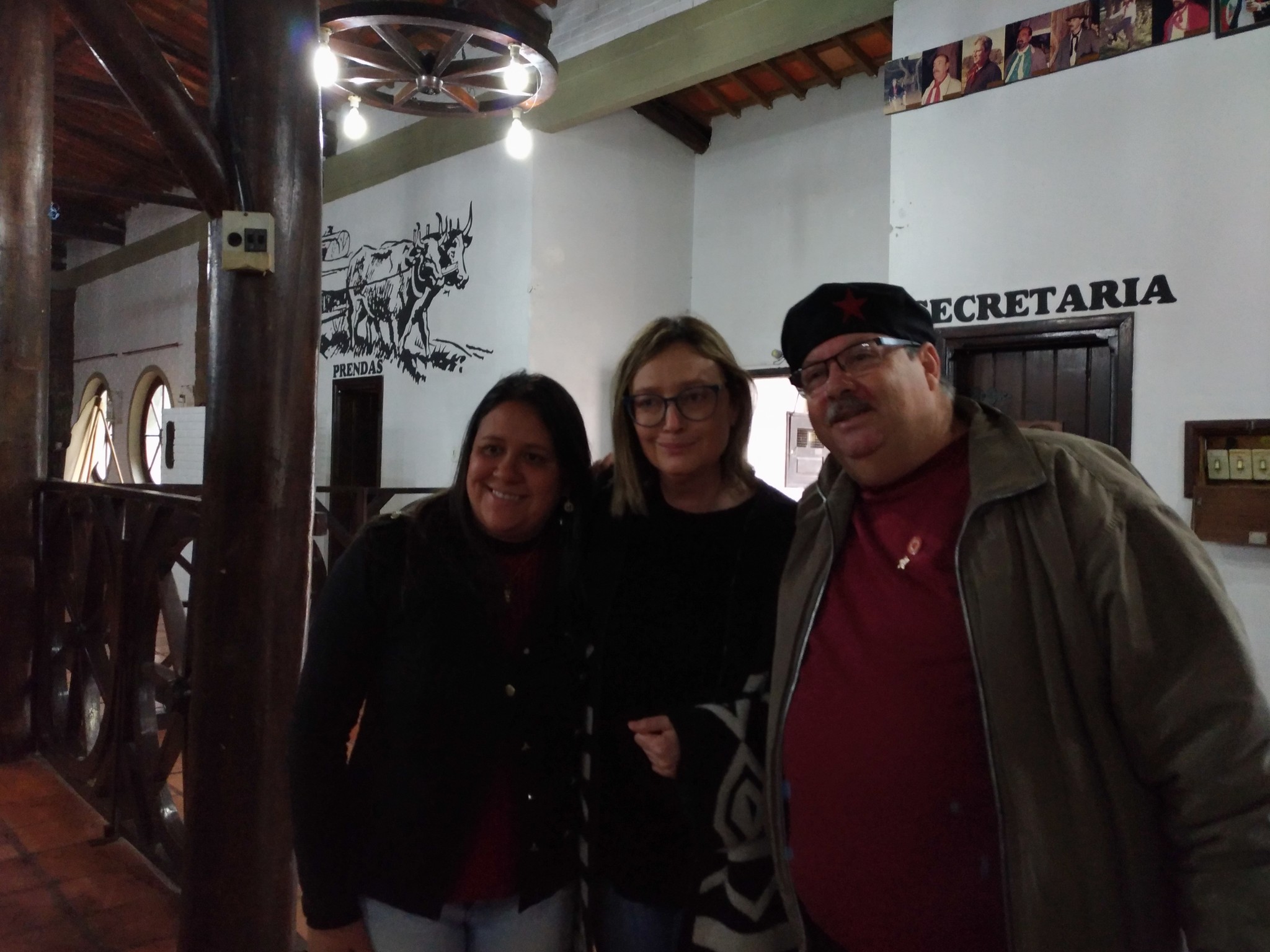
[782,282,935,376]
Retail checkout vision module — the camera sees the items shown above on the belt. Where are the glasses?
[789,335,920,392]
[623,383,737,428]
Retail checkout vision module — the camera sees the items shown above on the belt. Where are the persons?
[758,281,1270,952]
[588,315,798,952]
[288,369,598,952]
